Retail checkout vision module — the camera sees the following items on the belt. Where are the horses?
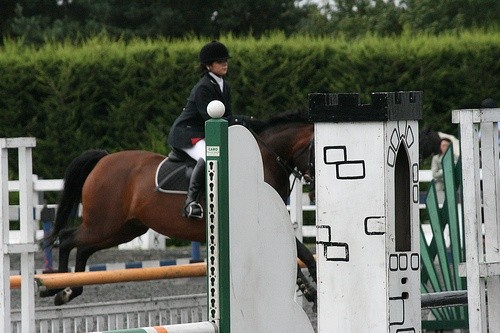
[38,108,315,306]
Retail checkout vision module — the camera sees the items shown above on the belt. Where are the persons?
[431,138,452,208]
[169,41,252,216]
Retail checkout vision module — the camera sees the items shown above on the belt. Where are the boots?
[180,158,205,215]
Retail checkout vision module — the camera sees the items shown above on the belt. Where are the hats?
[200,40,232,65]
